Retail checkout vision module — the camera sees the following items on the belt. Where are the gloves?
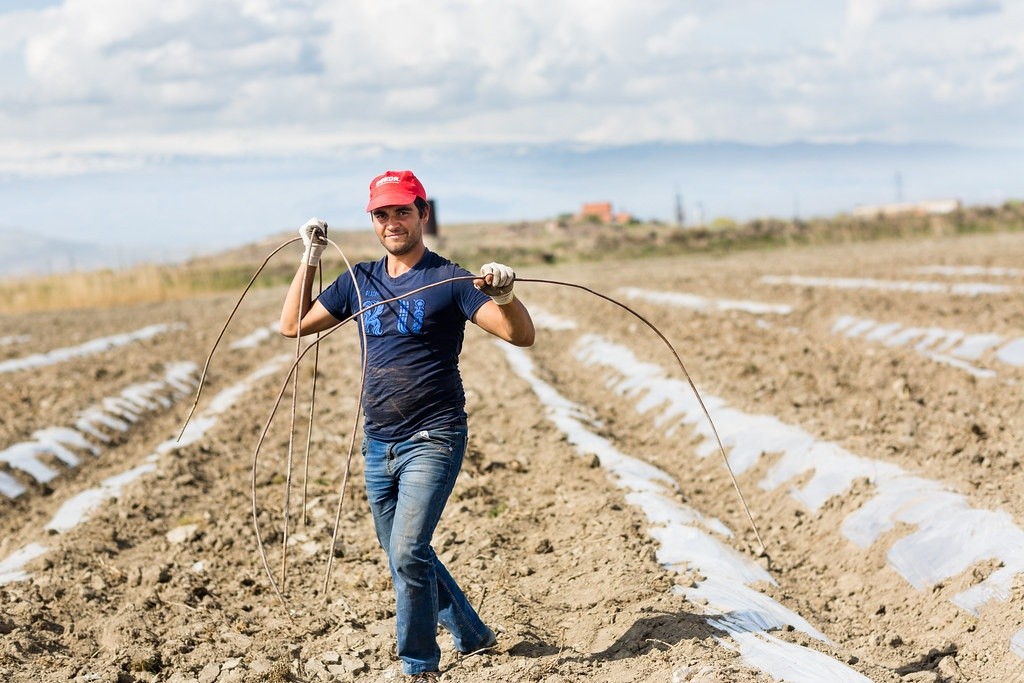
[300,219,330,267]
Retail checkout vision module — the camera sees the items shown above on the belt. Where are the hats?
[366,169,427,213]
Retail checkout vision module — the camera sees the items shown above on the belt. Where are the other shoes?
[454,632,496,655]
[408,674,442,683]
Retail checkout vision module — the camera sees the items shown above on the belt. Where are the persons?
[281,171,537,676]
